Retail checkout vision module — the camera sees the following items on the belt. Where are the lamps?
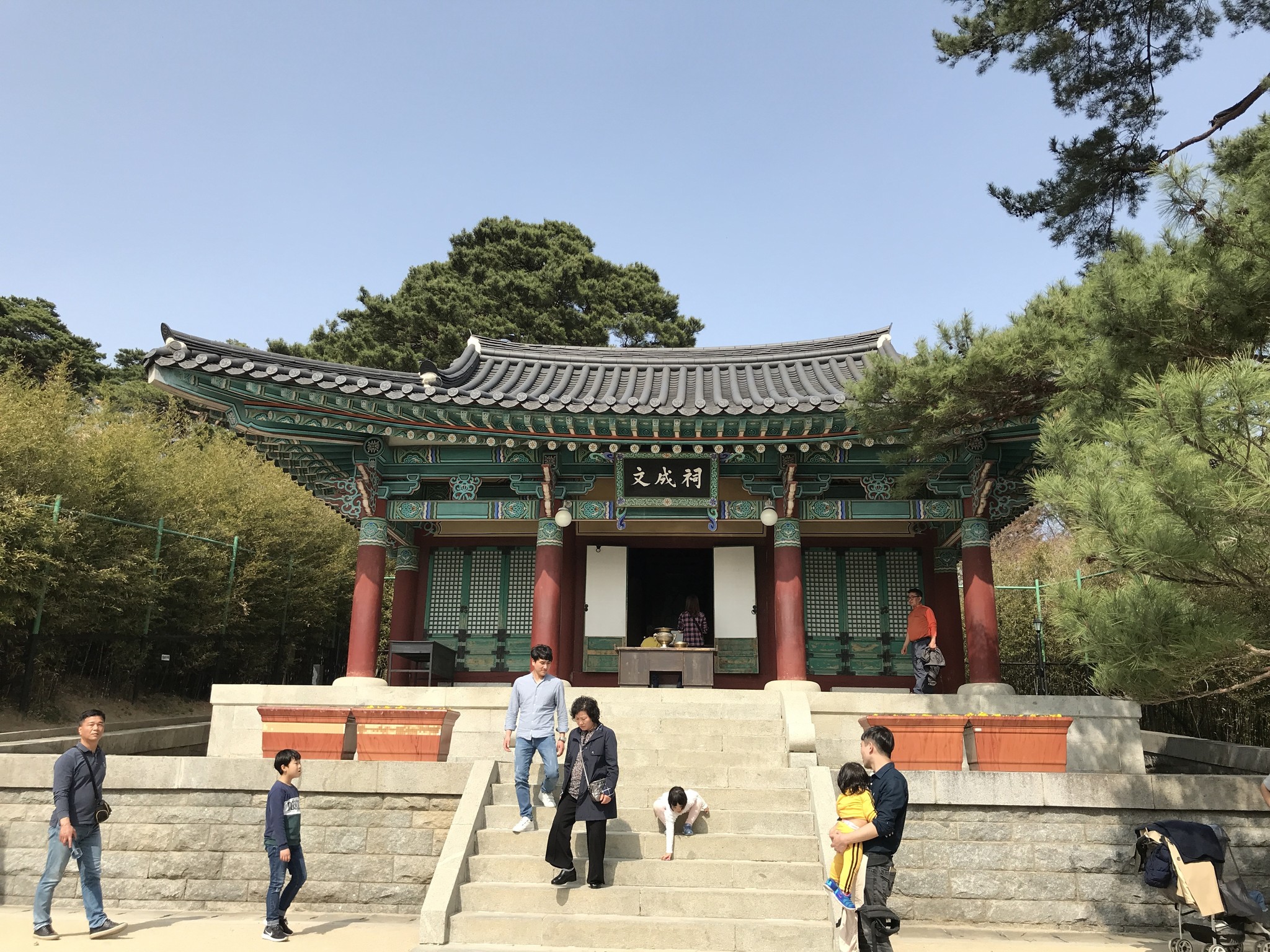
[554,499,572,527]
[760,499,778,526]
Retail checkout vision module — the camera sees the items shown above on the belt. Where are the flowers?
[964,711,1062,718]
[860,712,960,718]
[361,705,452,711]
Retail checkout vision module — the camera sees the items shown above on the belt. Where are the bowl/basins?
[673,641,688,648]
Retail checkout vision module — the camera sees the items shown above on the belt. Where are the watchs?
[558,737,567,743]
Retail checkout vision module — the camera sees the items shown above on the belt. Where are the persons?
[901,588,937,693]
[546,696,618,889]
[838,726,909,952]
[1260,773,1270,806]
[653,786,709,860]
[33,709,128,940]
[262,749,307,941]
[823,762,894,910]
[503,644,569,832]
[677,595,708,647]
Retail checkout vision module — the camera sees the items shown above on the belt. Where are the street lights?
[1033,618,1046,695]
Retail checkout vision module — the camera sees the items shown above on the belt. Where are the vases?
[969,716,1074,773]
[351,706,461,762]
[858,714,969,770]
[256,705,358,760]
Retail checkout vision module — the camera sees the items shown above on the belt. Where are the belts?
[912,636,931,643]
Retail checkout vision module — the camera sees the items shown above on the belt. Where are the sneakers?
[33,925,60,940]
[833,886,856,910]
[512,816,534,832]
[264,915,293,935]
[538,788,556,807]
[682,823,693,835]
[261,925,288,942]
[89,919,128,939]
[664,829,667,835]
[823,878,839,892]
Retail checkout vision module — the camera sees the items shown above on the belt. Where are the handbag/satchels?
[588,778,615,802]
[95,800,112,823]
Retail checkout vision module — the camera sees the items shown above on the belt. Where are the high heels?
[590,883,601,888]
[551,867,576,885]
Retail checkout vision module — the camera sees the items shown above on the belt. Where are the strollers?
[1134,820,1270,952]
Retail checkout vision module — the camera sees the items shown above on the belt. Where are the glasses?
[906,595,920,600]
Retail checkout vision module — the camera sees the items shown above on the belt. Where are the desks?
[614,643,720,689]
[388,640,457,687]
